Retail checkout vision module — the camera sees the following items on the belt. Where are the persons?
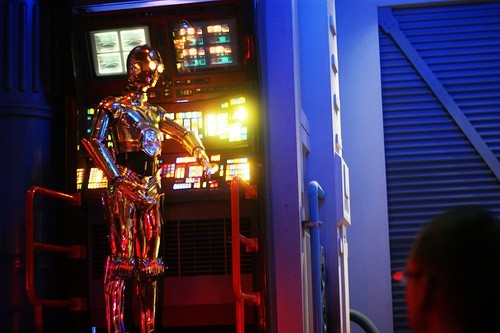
[405,205,500,333]
[78,45,212,333]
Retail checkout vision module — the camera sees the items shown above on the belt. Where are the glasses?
[400,267,440,286]
